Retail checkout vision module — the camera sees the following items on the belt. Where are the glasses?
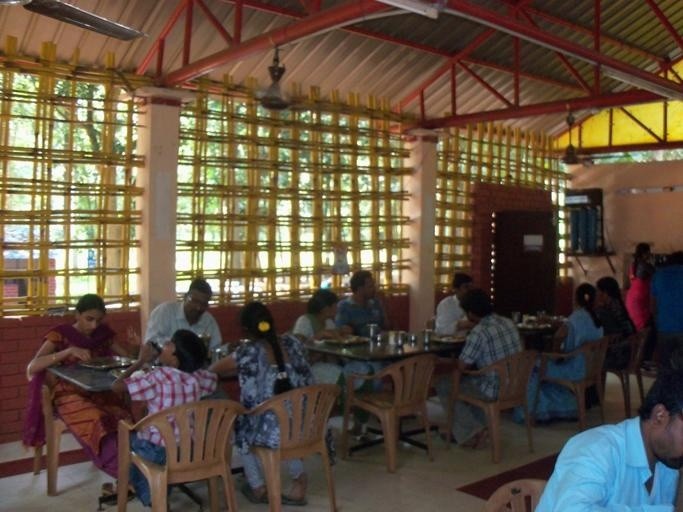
[187,292,209,308]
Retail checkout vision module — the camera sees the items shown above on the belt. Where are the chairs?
[600,326,652,420]
[529,336,612,433]
[116,399,246,512]
[26,359,69,496]
[481,477,547,512]
[246,383,341,512]
[444,349,547,464]
[341,352,440,473]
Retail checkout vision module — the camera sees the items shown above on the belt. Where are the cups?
[198,333,211,369]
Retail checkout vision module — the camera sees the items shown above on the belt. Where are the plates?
[110,365,157,377]
[431,341,467,344]
[79,355,135,369]
[325,334,369,349]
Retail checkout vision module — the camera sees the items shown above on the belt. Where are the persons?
[334,270,385,332]
[596,277,636,370]
[532,370,683,512]
[209,302,317,505]
[111,329,219,512]
[292,289,374,442]
[141,279,225,365]
[451,288,523,449]
[21,294,136,505]
[434,273,475,335]
[625,242,657,370]
[515,282,604,423]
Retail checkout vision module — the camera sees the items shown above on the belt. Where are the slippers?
[101,482,117,505]
[242,481,267,504]
[282,495,307,506]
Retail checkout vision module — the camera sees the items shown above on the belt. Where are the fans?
[533,114,631,165]
[0,0,148,42]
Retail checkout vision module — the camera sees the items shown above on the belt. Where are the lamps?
[259,47,292,110]
